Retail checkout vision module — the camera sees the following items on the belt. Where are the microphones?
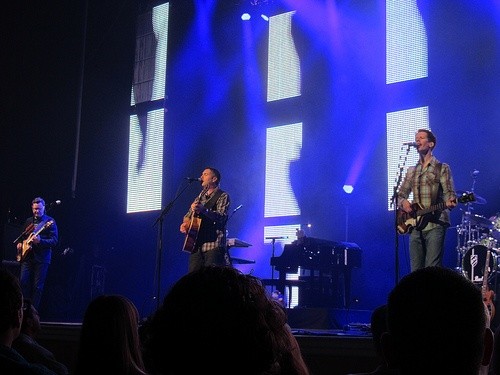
[186,177,202,181]
[46,200,61,205]
[404,142,419,147]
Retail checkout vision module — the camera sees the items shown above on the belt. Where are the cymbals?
[265,236,289,239]
[457,194,486,204]
[465,214,494,229]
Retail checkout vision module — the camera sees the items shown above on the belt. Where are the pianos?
[270,236,362,309]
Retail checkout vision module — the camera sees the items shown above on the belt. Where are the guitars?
[480,250,495,320]
[396,192,476,235]
[182,183,210,252]
[16,221,53,263]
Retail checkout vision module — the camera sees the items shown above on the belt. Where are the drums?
[455,224,479,251]
[477,226,489,240]
[461,211,475,225]
[461,245,500,284]
[480,235,497,249]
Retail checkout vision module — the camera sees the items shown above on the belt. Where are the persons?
[180,168,232,273]
[16,198,59,312]
[0,271,308,375]
[373,266,496,375]
[396,130,458,272]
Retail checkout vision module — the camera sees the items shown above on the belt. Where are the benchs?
[259,278,306,308]
